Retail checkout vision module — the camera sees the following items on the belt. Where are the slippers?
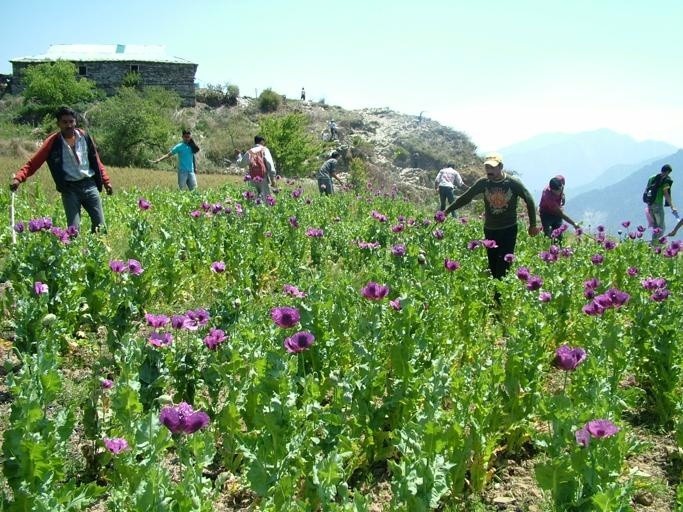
[248,147,267,181]
[643,174,671,205]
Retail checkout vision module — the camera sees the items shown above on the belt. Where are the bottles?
[483,153,503,168]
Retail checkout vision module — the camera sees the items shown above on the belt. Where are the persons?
[299,86,307,100]
[432,151,538,282]
[641,162,678,249]
[146,130,198,193]
[315,150,344,199]
[326,117,337,141]
[432,162,471,221]
[234,134,278,197]
[6,103,115,239]
[537,174,581,244]
